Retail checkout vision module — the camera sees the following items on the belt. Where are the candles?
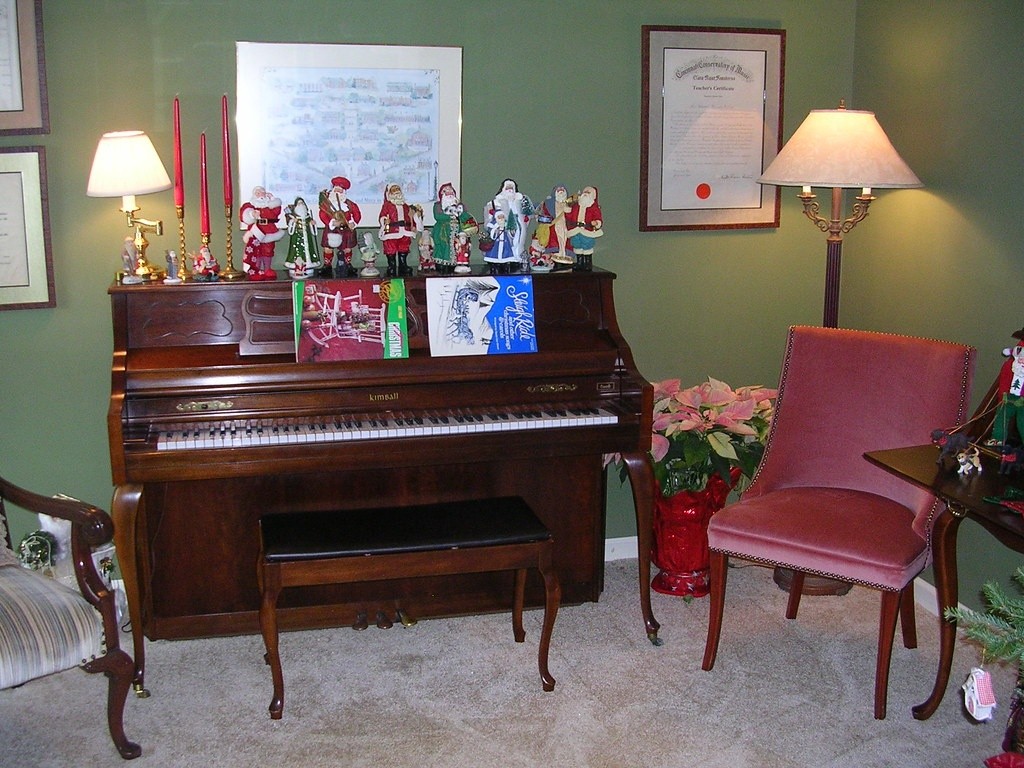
[222,93,233,205]
[200,127,211,234]
[173,90,184,206]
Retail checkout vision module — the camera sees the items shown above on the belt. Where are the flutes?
[318,193,348,231]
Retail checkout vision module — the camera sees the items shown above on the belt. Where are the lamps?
[755,98,928,597]
[86,131,173,282]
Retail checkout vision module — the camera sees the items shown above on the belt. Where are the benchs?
[254,496,561,720]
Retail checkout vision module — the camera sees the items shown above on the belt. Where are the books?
[426,275,538,357]
[292,278,409,362]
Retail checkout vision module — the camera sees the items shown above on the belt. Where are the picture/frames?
[235,41,464,228]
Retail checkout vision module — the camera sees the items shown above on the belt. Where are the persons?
[984,327,1024,446]
[167,250,178,279]
[240,186,285,280]
[193,246,219,278]
[378,182,413,275]
[483,177,535,274]
[285,198,321,275]
[319,176,361,274]
[530,184,603,270]
[121,237,139,276]
[417,183,479,273]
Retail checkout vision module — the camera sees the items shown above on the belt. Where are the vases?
[651,468,743,598]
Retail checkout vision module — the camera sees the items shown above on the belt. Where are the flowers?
[601,376,779,497]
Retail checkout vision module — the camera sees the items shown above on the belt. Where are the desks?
[862,442,1024,720]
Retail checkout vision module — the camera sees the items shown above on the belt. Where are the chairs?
[702,326,979,720]
[0,478,142,759]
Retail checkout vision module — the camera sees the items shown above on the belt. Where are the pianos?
[107,260,661,700]
